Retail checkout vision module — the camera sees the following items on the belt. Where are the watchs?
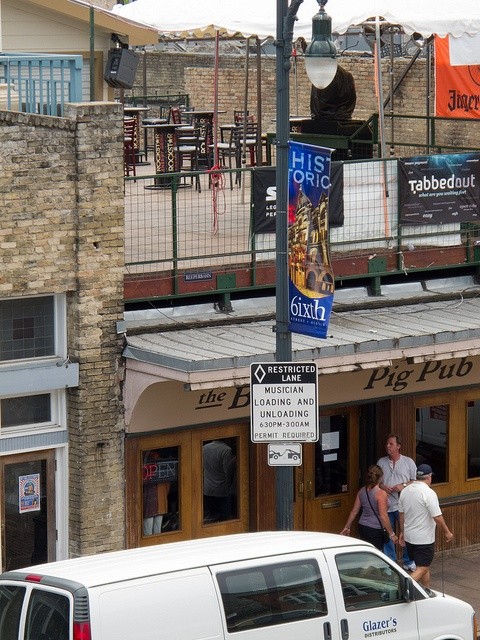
[401,483,407,488]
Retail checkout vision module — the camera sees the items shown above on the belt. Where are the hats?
[417,464,434,476]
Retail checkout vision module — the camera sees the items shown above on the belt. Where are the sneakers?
[403,561,416,571]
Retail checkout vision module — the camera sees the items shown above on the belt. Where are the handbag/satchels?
[383,528,391,543]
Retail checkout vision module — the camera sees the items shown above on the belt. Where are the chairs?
[178,106,195,126]
[172,106,182,124]
[172,126,202,192]
[235,113,255,165]
[177,121,211,170]
[236,121,272,166]
[141,106,171,162]
[124,119,137,182]
[208,125,244,191]
[219,110,249,167]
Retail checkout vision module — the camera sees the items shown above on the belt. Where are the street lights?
[272,0,351,532]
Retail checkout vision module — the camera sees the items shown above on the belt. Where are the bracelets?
[388,533,396,539]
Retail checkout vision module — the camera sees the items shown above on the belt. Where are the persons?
[340,463,399,552]
[399,463,453,587]
[227,453,237,517]
[203,439,232,519]
[377,433,418,572]
[142,451,170,536]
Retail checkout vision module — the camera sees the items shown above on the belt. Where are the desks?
[141,122,192,190]
[124,107,151,166]
[182,110,227,169]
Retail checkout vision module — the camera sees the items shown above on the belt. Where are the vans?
[0,529,479,640]
